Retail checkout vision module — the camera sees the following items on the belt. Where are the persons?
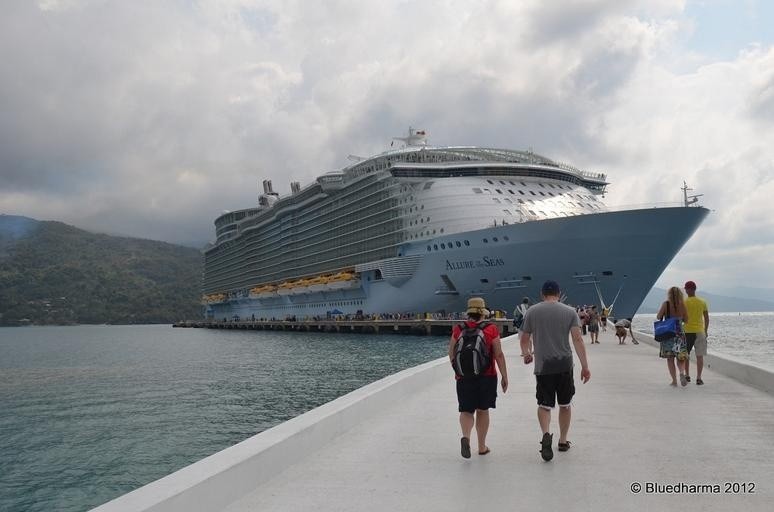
[519,280,591,461]
[681,280,709,385]
[223,309,508,322]
[513,295,535,356]
[447,297,508,458]
[656,288,689,386]
[615,318,633,344]
[567,304,609,344]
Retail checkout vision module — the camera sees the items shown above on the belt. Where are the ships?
[195,123,719,328]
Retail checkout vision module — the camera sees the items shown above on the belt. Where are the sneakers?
[460,437,471,459]
[539,432,555,462]
[669,376,704,387]
[588,340,631,346]
[557,439,572,452]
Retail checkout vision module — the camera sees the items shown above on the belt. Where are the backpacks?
[450,320,496,378]
[513,303,528,330]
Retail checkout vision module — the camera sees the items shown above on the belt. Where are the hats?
[466,297,494,317]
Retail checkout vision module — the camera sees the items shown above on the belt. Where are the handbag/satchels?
[653,300,680,343]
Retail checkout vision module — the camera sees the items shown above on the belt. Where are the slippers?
[478,446,491,455]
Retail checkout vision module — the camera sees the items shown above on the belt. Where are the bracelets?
[520,352,529,357]
